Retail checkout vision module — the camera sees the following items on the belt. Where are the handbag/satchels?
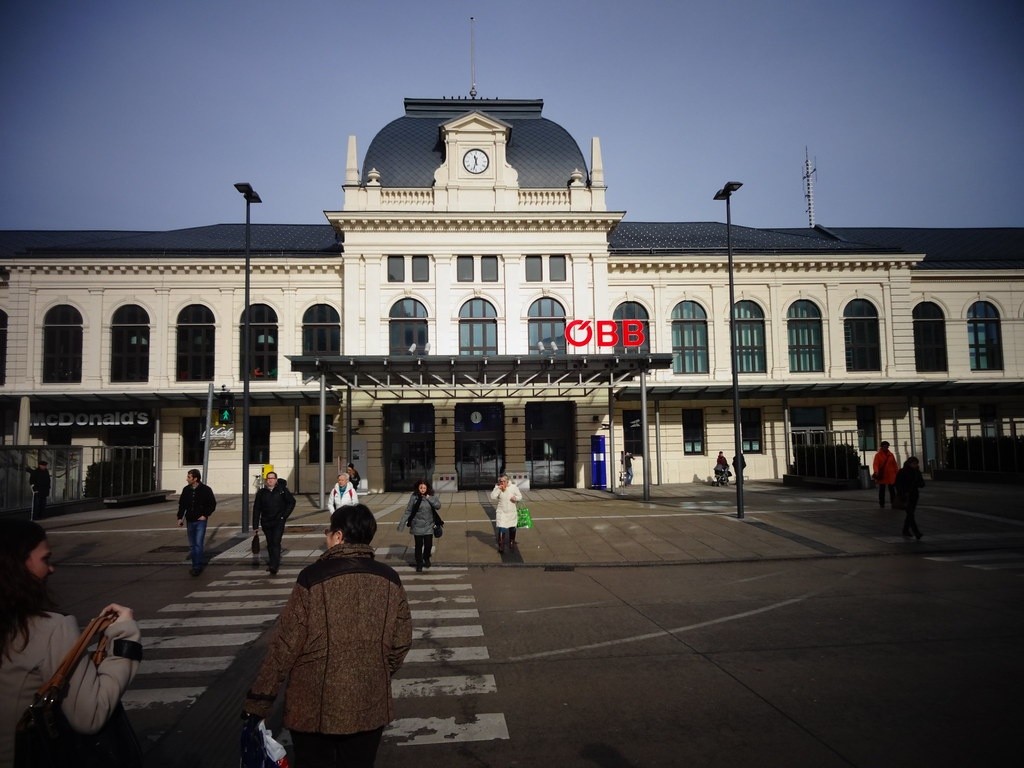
[872,470,883,481]
[14,615,145,768]
[517,498,533,528]
[241,719,276,768]
[252,530,259,554]
[258,720,291,767]
[434,526,443,538]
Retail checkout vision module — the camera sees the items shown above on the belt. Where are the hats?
[347,463,354,469]
[38,461,47,465]
[266,472,277,479]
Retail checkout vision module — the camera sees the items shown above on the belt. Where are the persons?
[327,472,358,515]
[176,470,216,576]
[397,480,441,572]
[240,503,412,768]
[896,457,925,540]
[346,463,360,490]
[717,451,727,485]
[252,472,295,574]
[873,441,899,508]
[624,450,634,486]
[732,449,746,484]
[490,473,522,554]
[0,518,143,768]
[29,461,51,520]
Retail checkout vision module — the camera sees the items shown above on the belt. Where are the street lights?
[232,182,263,533]
[712,180,745,520]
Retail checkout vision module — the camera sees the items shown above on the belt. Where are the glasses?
[325,527,336,536]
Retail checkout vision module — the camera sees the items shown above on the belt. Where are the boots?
[499,533,504,553]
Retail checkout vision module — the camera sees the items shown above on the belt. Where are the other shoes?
[916,533,923,540]
[903,528,913,536]
[186,550,192,560]
[423,555,430,567]
[191,566,203,576]
[509,529,515,549]
[266,566,278,575]
[416,561,423,572]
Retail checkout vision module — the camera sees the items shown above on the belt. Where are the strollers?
[713,464,730,487]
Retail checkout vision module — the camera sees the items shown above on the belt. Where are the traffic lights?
[216,391,235,425]
